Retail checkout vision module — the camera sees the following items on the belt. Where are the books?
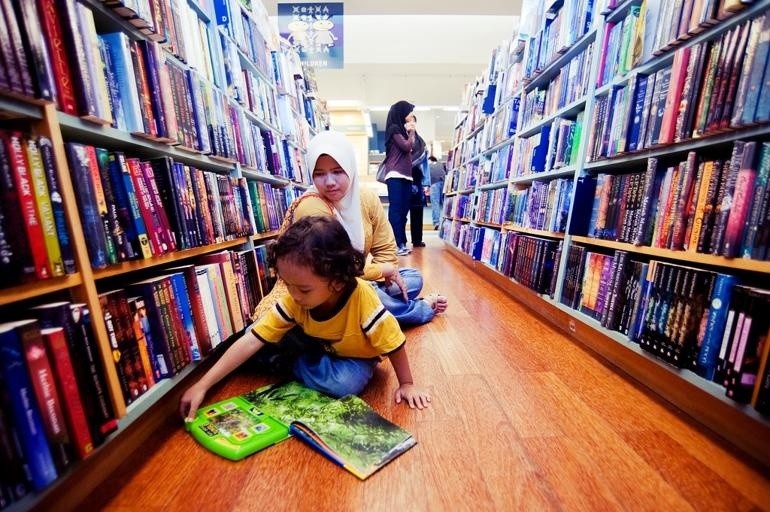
[239,377,418,482]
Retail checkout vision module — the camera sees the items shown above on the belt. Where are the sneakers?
[403,247,413,253]
[413,241,425,248]
[433,223,440,230]
[396,249,407,256]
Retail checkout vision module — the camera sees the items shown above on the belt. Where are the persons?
[428,155,446,230]
[179,215,431,411]
[407,116,431,248]
[384,100,415,257]
[249,130,449,329]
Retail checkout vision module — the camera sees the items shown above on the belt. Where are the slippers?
[417,293,443,315]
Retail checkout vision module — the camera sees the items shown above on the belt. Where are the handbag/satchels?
[375,159,388,185]
[247,275,291,322]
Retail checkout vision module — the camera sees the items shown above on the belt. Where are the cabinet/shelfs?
[0,0,327,509]
[440,1,770,470]
